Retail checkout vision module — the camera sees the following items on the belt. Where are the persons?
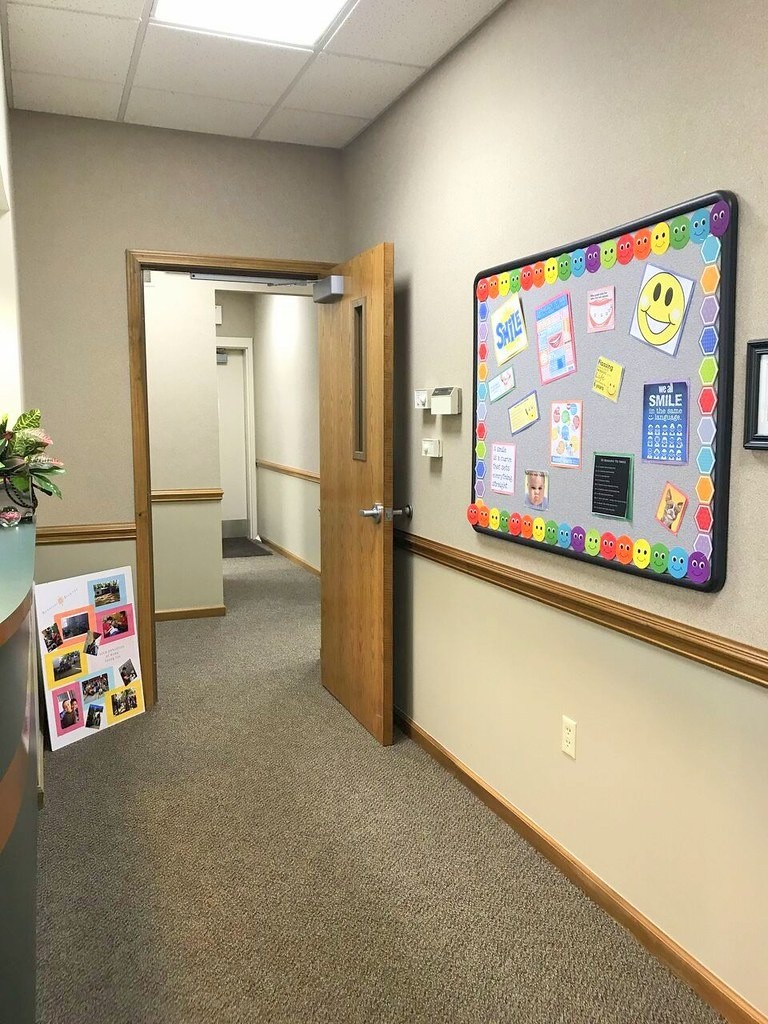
[85,676,108,696]
[524,471,548,511]
[102,610,128,638]
[88,640,95,655]
[113,695,136,716]
[43,627,60,650]
[61,698,79,729]
[120,667,134,686]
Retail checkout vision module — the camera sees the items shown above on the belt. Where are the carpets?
[222,537,274,558]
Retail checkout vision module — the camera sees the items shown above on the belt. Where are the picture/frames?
[743,339,768,450]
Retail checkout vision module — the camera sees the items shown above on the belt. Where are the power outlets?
[562,716,577,760]
